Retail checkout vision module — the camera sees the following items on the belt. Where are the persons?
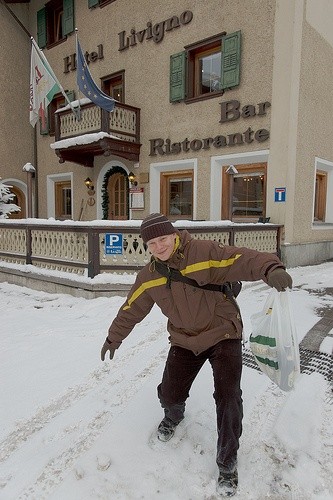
[101,213,292,498]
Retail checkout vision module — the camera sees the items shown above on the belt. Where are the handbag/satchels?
[150,255,242,298]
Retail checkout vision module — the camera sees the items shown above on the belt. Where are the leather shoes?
[216,468,239,500]
[155,413,178,441]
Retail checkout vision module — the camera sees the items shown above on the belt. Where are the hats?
[140,213,176,243]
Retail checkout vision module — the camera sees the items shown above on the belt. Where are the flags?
[29,27,116,129]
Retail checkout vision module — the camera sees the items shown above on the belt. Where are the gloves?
[267,267,293,292]
[101,337,116,361]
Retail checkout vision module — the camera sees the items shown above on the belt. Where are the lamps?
[84,177,94,190]
[128,171,137,186]
